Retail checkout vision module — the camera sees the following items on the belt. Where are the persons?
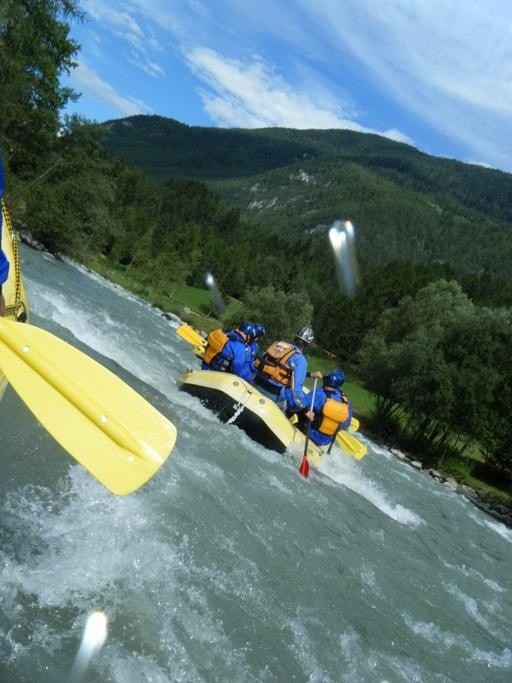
[301,368,352,445]
[201,320,257,382]
[252,326,322,421]
[223,322,266,358]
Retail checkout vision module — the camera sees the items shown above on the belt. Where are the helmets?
[240,321,265,340]
[322,369,345,387]
[295,327,315,344]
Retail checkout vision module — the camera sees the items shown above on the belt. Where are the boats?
[169,361,325,472]
[1,195,31,411]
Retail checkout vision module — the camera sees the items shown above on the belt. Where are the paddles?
[1,317,178,496]
[335,429,367,460]
[350,416,359,431]
[299,378,317,478]
[176,324,208,349]
